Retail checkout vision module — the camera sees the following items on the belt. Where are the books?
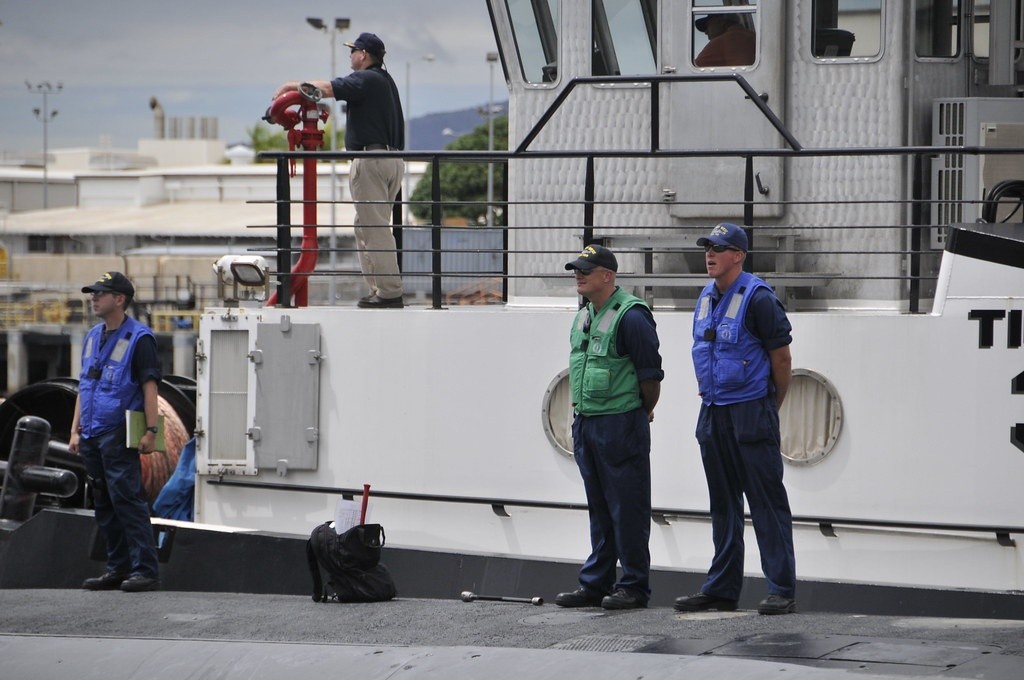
[125,409,165,451]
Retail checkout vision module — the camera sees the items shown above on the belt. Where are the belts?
[359,141,402,151]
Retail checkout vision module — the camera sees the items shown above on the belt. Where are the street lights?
[402,53,436,232]
[24,80,66,208]
[306,16,352,304]
[485,52,501,228]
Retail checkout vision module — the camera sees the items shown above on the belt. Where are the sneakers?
[121,574,162,593]
[757,592,796,617]
[84,572,122,590]
[673,590,739,613]
[554,585,601,608]
[601,587,651,611]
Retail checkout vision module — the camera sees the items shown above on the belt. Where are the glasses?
[351,47,362,53]
[92,291,118,299]
[702,242,741,253]
[570,267,605,276]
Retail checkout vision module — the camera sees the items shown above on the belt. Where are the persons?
[556,245,664,609]
[696,13,754,66]
[69,272,161,591]
[272,32,403,309]
[673,224,796,615]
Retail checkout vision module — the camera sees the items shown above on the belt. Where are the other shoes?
[357,293,405,309]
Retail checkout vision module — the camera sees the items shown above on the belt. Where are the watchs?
[147,426,158,432]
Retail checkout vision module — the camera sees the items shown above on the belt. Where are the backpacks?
[305,521,398,604]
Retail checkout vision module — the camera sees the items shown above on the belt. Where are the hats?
[81,271,135,298]
[696,223,748,253]
[696,14,741,30]
[565,244,618,275]
[342,33,386,59]
